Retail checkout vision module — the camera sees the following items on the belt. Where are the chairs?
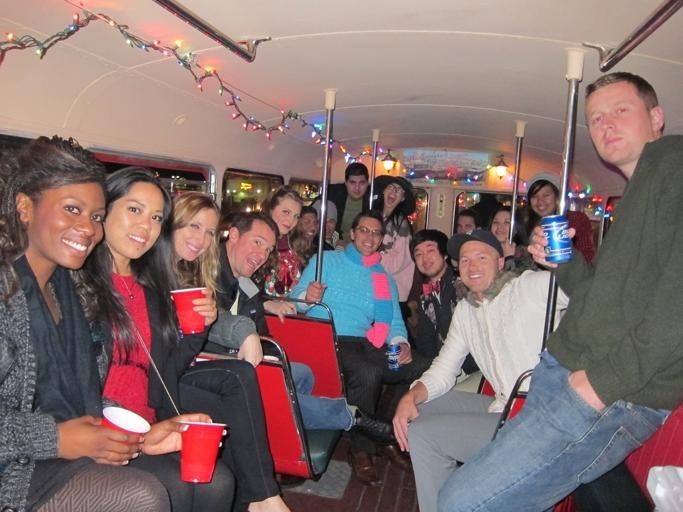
[478,373,495,401]
[454,366,574,512]
[190,332,346,484]
[257,293,348,403]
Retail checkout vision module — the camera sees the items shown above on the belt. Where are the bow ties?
[421,281,442,298]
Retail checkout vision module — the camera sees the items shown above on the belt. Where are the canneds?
[385,343,401,371]
[541,214,574,264]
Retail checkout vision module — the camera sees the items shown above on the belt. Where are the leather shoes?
[348,444,378,485]
[381,444,412,471]
[346,407,397,443]
[274,473,306,489]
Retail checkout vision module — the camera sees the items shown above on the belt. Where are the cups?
[95,408,151,445]
[168,287,205,335]
[177,420,227,484]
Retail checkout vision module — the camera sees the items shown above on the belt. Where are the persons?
[311,163,388,255]
[76,166,236,512]
[287,207,321,273]
[455,211,481,236]
[286,210,412,487]
[139,189,294,512]
[390,229,569,512]
[371,177,418,303]
[261,184,301,323]
[0,134,227,512]
[437,71,682,512]
[310,198,347,251]
[217,210,398,440]
[490,206,528,270]
[527,171,597,264]
[386,229,455,421]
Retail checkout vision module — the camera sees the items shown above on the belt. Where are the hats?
[447,229,504,261]
[310,199,338,221]
[368,175,416,216]
[527,172,561,195]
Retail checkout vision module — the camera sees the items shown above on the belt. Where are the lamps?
[381,149,395,172]
[494,154,508,178]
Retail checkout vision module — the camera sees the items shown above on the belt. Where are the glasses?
[354,228,382,236]
